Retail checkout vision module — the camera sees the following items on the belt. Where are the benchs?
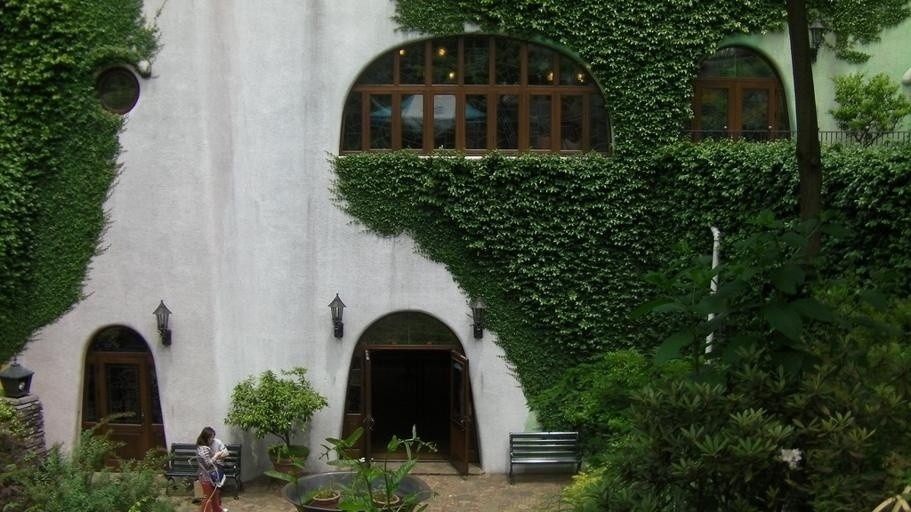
[163,443,245,501]
[505,430,583,486]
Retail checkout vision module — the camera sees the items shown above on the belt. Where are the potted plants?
[312,428,439,510]
[223,365,332,487]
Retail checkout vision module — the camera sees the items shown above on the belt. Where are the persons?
[195,427,229,512]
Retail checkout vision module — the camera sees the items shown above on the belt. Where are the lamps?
[152,299,173,348]
[327,294,346,339]
[469,294,488,339]
